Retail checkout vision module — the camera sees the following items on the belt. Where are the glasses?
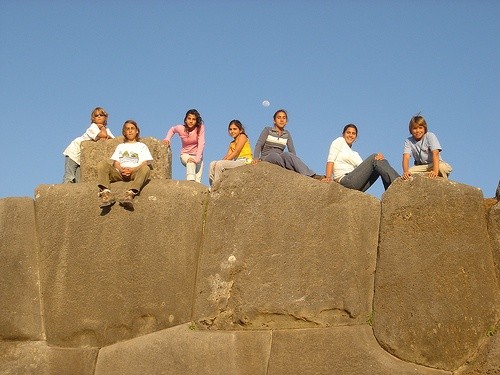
[94,114,105,117]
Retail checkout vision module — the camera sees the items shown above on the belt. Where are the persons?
[401,112,453,181]
[96,119,154,208]
[62,106,115,183]
[251,109,326,183]
[320,123,401,192]
[162,109,205,183]
[208,120,252,191]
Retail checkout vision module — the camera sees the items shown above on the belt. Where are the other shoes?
[315,175,326,180]
[119,191,135,209]
[99,189,116,208]
